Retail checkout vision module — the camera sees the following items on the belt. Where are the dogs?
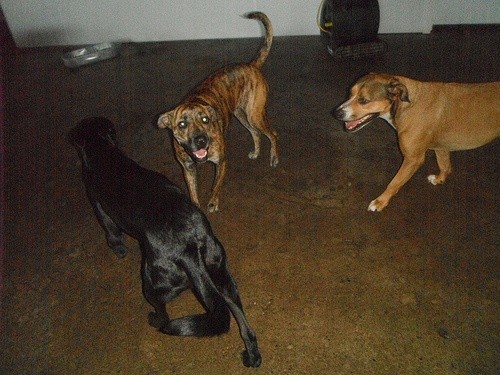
[334,71,500,212]
[67,116,263,369]
[157,12,279,213]
